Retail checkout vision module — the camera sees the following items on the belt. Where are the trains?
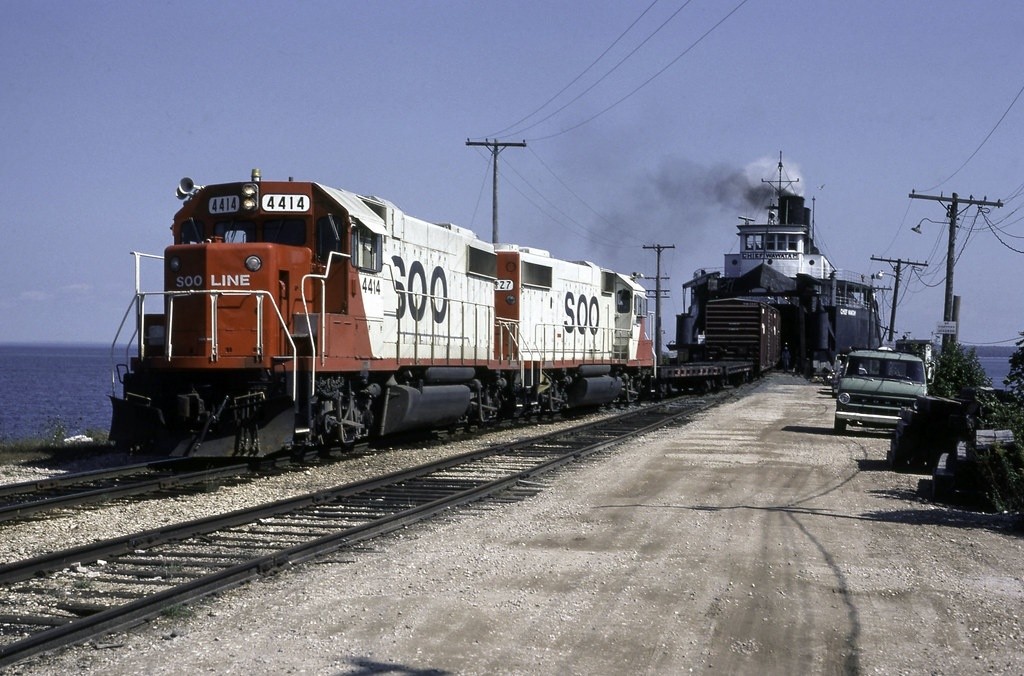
[108,166,883,467]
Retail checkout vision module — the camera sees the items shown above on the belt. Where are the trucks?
[833,347,937,437]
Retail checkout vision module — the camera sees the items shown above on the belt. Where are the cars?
[831,365,868,399]
